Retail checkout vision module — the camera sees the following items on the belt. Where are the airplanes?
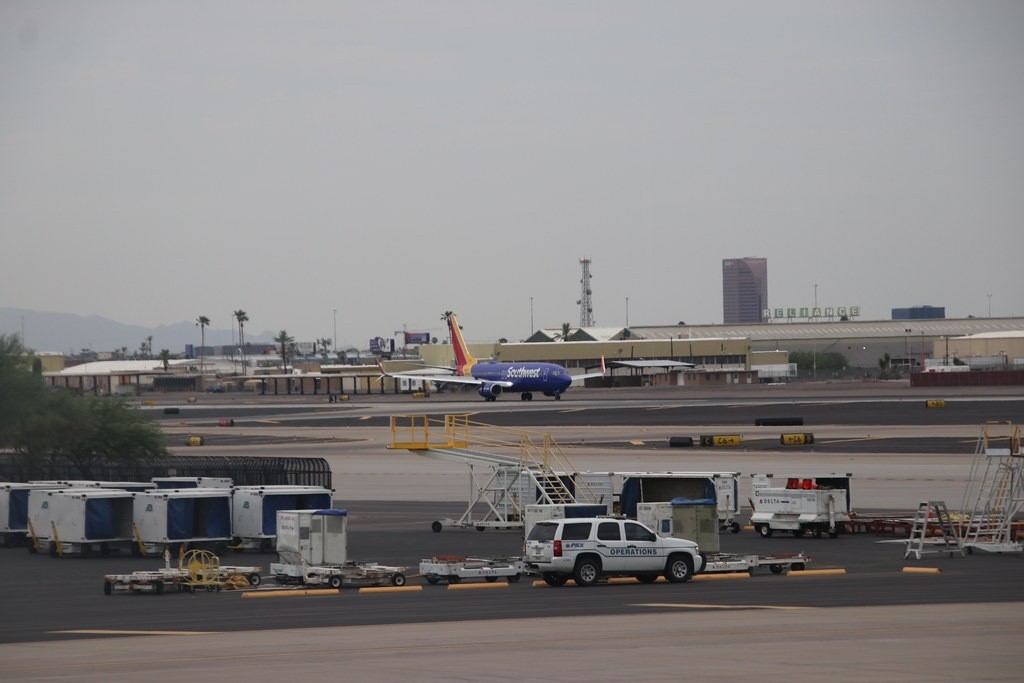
[374,310,606,403]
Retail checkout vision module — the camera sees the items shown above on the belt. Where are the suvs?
[525,515,707,587]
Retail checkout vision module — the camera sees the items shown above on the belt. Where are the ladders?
[957,419,1024,553]
[905,499,966,560]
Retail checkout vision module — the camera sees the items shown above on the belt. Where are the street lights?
[987,293,993,318]
[905,329,912,373]
